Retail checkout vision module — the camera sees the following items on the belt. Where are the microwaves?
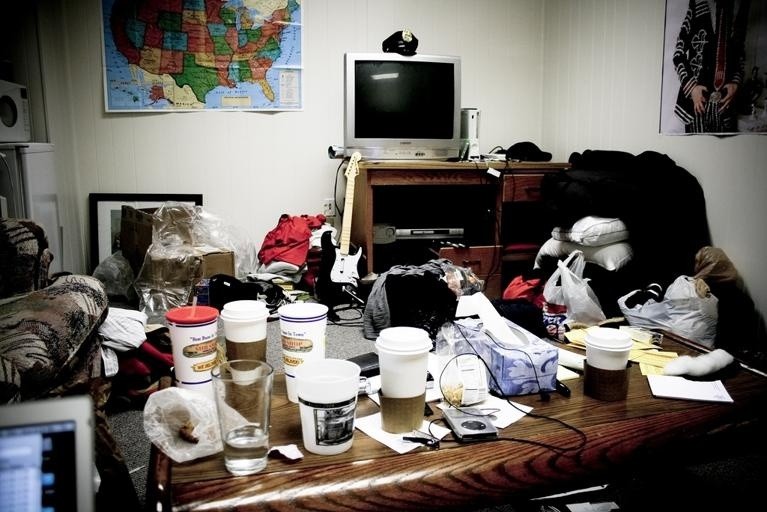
[0,80,32,143]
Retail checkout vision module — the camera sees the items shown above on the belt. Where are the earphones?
[430,323,587,451]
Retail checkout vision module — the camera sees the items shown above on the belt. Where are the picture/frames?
[89,193,202,272]
[659,0,767,135]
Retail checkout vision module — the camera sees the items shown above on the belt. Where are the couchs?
[0,220,108,403]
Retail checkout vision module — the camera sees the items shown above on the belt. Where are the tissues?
[455,292,559,395]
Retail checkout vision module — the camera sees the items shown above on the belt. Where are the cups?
[211,359,272,478]
[294,358,363,456]
[585,324,633,400]
[276,302,330,404]
[376,326,434,432]
[220,300,269,386]
[165,306,218,389]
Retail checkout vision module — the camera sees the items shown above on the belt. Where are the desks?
[151,323,724,512]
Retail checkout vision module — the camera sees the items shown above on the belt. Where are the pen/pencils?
[557,380,571,395]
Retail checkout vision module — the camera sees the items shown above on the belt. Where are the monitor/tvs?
[1,395,100,512]
[342,53,463,163]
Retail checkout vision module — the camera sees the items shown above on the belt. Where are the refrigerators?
[0,143,63,278]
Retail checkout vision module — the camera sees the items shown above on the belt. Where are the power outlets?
[321,200,336,217]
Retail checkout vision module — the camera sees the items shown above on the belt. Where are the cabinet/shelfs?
[348,162,573,295]
